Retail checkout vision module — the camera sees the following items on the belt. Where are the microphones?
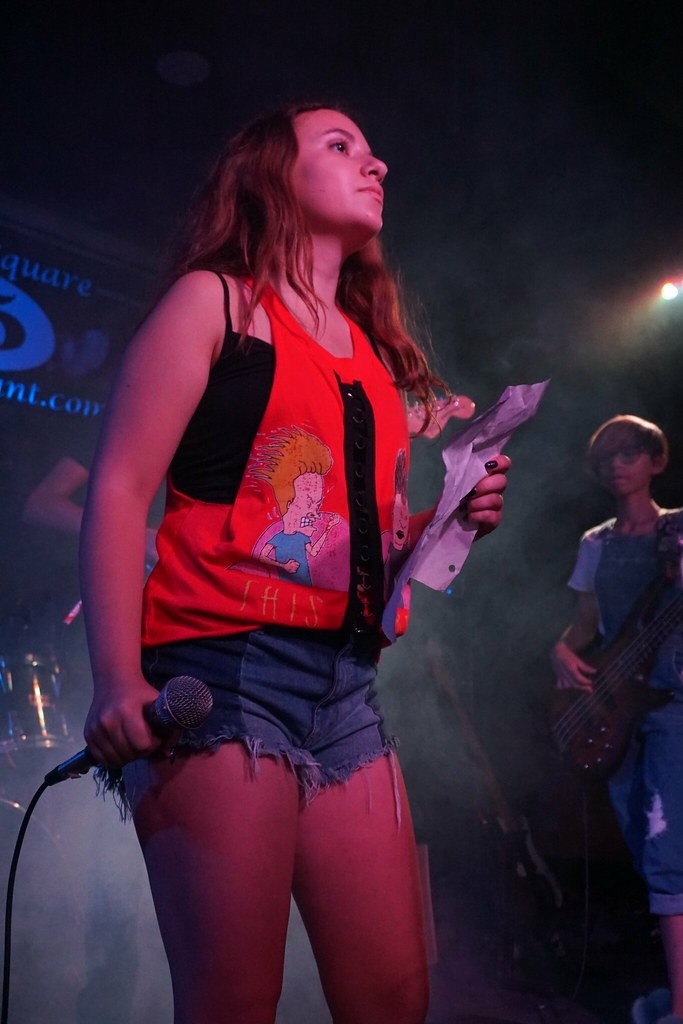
[42,676,213,787]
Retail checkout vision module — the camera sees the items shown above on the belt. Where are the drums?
[0,650,73,760]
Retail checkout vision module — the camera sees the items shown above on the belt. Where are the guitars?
[544,590,683,786]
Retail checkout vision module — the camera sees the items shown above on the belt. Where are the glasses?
[597,445,645,467]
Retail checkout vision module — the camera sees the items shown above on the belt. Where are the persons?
[22,99,513,1023]
[550,412,683,1024]
